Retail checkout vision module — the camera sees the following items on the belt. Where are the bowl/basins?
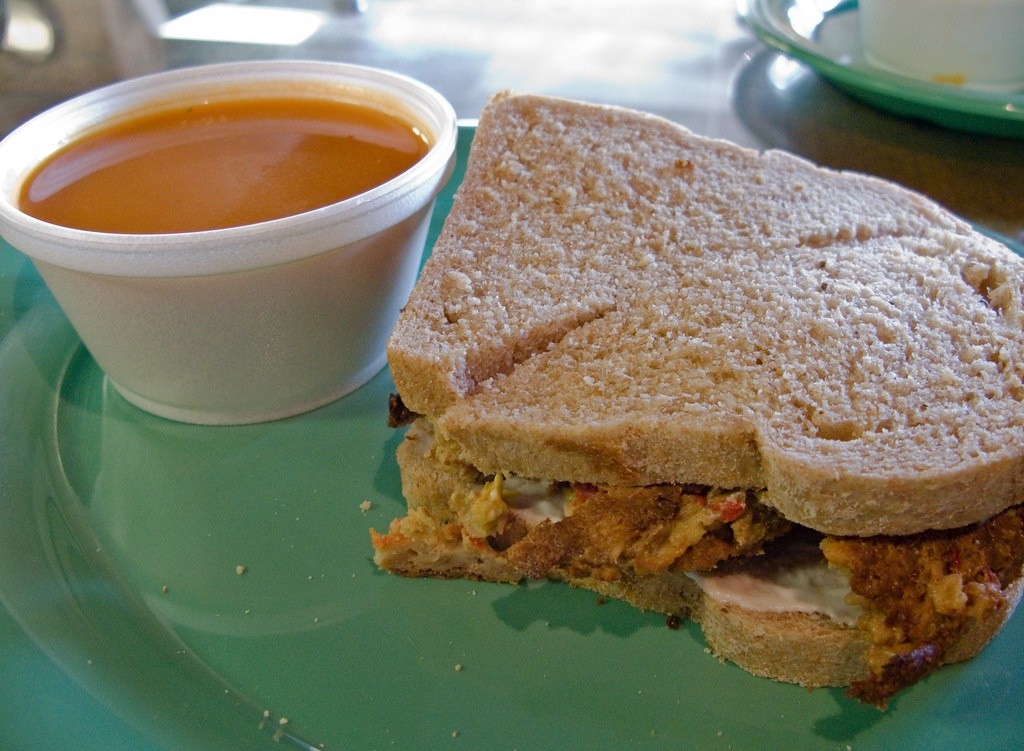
[1,60,457,426]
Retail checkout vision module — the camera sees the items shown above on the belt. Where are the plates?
[736,1,1024,140]
[0,120,1024,751]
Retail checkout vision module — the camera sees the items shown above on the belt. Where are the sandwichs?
[356,91,1024,692]
